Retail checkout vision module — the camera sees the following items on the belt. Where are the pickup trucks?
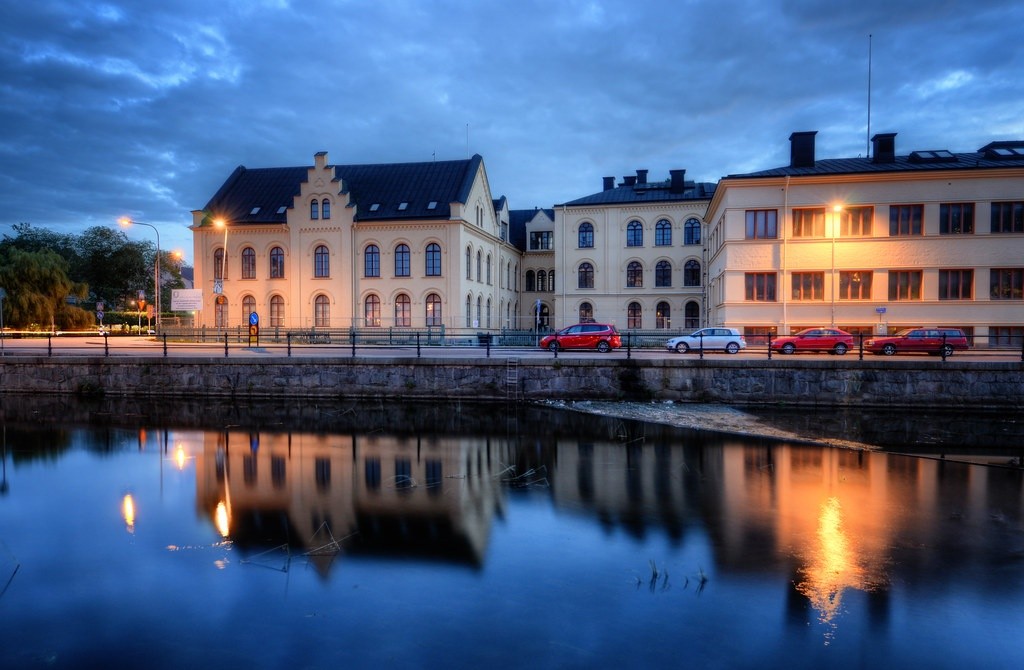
[863,327,969,358]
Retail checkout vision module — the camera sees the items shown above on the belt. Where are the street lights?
[120,219,162,326]
[215,220,229,342]
[830,205,842,328]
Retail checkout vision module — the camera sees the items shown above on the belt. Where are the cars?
[770,327,853,356]
[667,328,746,354]
[539,323,622,353]
